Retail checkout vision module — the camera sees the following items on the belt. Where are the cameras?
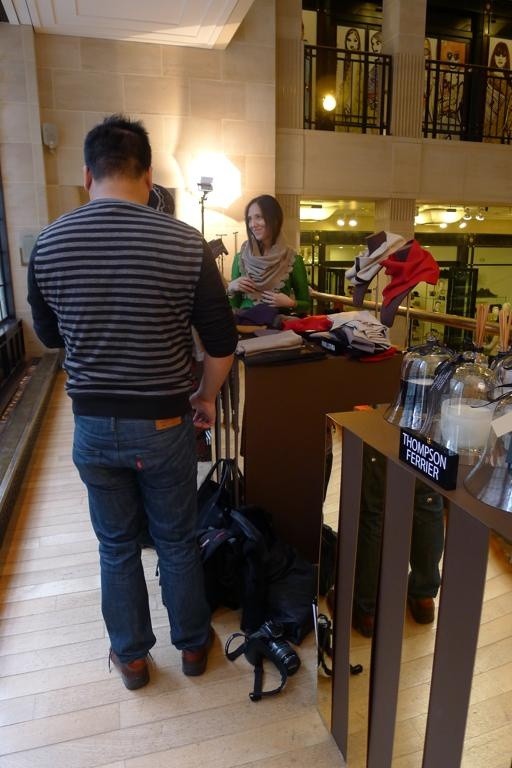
[317,614,331,666]
[244,614,300,676]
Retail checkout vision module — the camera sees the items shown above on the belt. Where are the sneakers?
[182,627,216,675]
[410,598,436,624]
[108,645,153,691]
[325,589,375,638]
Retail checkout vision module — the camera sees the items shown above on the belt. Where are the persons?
[26,112,239,690]
[438,41,464,140]
[365,31,383,136]
[326,440,447,638]
[483,40,511,143]
[424,37,437,138]
[135,180,176,552]
[226,193,314,312]
[334,27,361,133]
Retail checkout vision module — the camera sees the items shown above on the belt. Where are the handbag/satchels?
[195,457,275,610]
